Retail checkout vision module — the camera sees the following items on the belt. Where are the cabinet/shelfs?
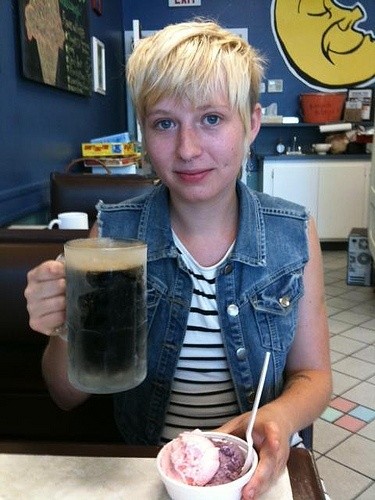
[259,158,370,244]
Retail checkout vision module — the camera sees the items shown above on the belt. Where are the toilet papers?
[318,122,352,133]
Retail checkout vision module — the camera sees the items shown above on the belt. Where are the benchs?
[0,170,313,448]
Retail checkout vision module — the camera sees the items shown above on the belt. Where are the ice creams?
[160,429,247,487]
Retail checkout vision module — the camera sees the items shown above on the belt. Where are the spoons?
[238,352,271,478]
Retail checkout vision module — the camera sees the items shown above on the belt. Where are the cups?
[54,237,147,395]
[156,432,258,500]
[48,212,89,231]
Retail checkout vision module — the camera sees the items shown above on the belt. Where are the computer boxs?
[347,227,372,286]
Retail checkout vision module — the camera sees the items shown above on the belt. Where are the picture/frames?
[92,36,107,96]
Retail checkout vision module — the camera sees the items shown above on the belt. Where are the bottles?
[345,98,371,121]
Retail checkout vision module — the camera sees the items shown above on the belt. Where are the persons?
[24,16,335,500]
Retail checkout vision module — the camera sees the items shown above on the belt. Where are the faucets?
[292,136,297,155]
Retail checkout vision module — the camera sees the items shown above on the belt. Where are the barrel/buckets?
[299,92,346,124]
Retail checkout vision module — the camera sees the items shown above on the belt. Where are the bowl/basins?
[312,143,332,154]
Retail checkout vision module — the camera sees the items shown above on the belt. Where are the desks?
[0,438,326,500]
